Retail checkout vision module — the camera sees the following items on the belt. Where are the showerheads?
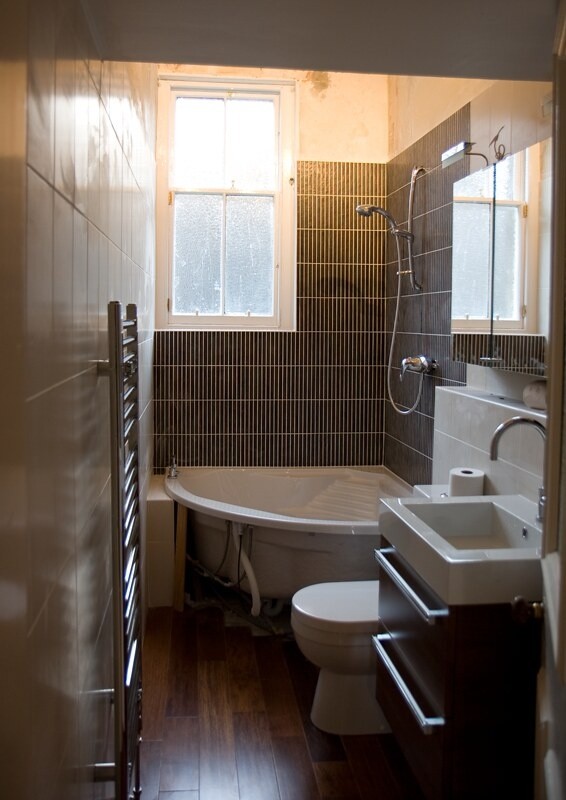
[356,203,399,232]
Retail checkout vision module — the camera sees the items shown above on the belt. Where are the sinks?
[376,496,546,607]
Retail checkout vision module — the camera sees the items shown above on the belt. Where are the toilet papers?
[449,466,485,495]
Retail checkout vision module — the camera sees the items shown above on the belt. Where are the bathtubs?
[162,463,414,601]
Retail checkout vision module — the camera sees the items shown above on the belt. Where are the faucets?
[487,414,548,523]
[168,464,180,479]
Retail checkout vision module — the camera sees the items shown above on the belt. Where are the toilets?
[291,579,394,735]
